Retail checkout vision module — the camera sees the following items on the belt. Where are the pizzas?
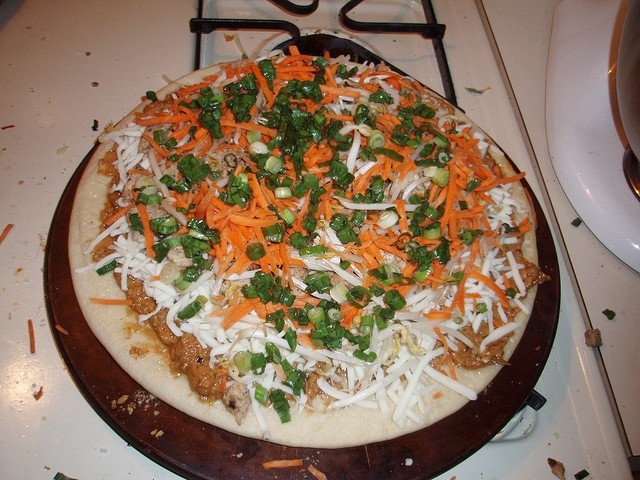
[66,57,539,450]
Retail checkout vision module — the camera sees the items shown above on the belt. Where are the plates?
[43,121,561,479]
